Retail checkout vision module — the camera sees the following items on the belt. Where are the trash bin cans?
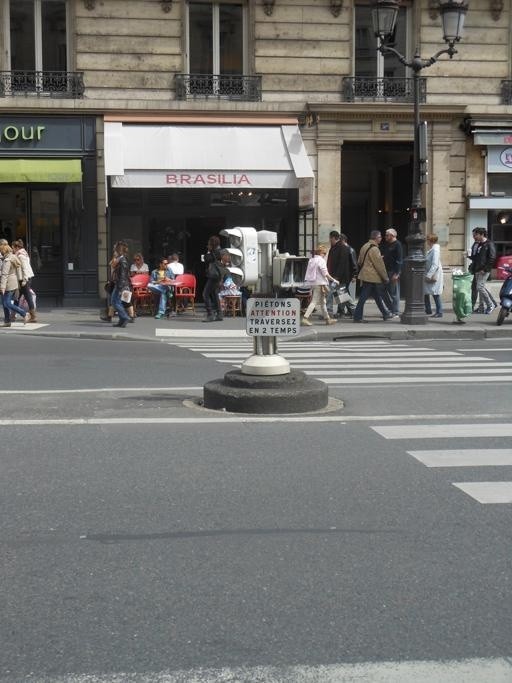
[451,272,472,318]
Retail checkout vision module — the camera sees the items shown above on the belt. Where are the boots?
[0,311,36,327]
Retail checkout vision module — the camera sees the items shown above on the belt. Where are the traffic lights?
[219,228,244,284]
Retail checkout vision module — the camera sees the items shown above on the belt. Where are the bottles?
[331,279,336,288]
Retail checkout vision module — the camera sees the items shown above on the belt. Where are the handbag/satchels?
[19,288,36,312]
[105,280,115,294]
[205,250,221,279]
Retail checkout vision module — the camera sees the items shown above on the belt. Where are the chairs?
[496,255,512,280]
[130,273,197,317]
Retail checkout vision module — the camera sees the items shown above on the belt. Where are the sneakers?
[474,303,495,313]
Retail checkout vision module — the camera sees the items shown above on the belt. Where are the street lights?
[371,0,469,326]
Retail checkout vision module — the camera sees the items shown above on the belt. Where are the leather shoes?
[113,318,131,327]
[326,319,337,325]
[302,320,312,325]
[202,315,223,321]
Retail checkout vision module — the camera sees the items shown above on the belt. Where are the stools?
[294,294,312,318]
[224,295,244,318]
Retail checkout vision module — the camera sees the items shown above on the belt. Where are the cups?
[466,248,473,255]
[201,254,205,261]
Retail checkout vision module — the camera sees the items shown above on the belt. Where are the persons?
[423,234,444,317]
[0,237,39,327]
[466,229,498,312]
[300,230,357,326]
[380,229,402,317]
[352,230,395,323]
[476,228,496,314]
[100,241,186,327]
[201,237,224,323]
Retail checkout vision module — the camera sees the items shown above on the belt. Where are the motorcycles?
[497,262,512,324]
